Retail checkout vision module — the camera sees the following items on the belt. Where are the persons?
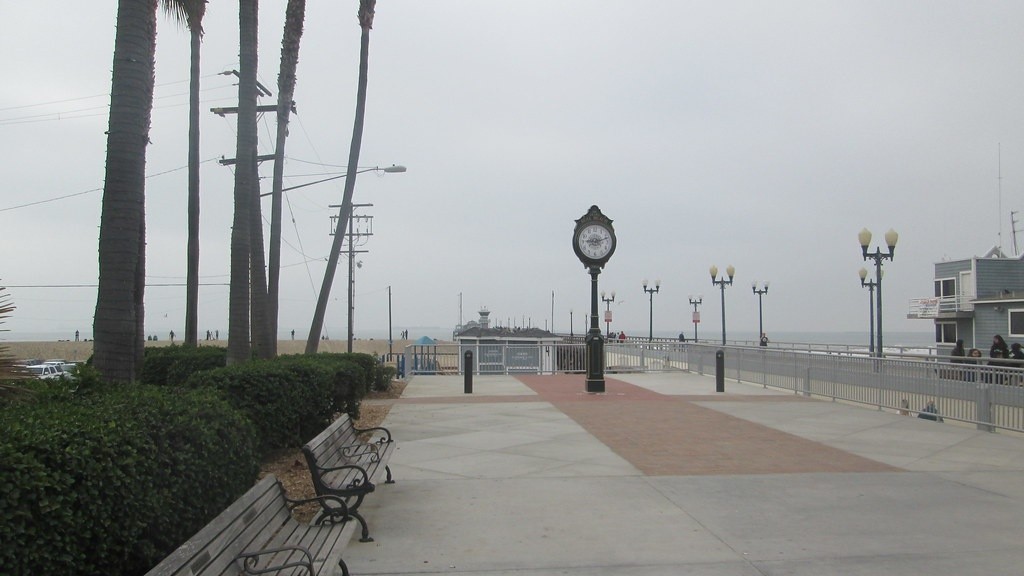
[405,330,408,339]
[215,330,218,338]
[76,330,79,341]
[760,333,768,346]
[148,335,152,340]
[206,330,210,340]
[169,330,176,341]
[322,336,324,340]
[962,349,981,382]
[950,339,965,363]
[291,329,295,340]
[982,335,1024,385]
[917,401,944,422]
[896,400,911,416]
[153,335,157,340]
[609,331,626,343]
[679,333,685,342]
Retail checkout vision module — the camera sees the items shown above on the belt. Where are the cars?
[12,358,87,383]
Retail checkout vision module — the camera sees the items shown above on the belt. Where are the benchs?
[142,464,358,576]
[301,409,396,542]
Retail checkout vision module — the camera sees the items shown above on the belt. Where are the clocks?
[578,224,613,260]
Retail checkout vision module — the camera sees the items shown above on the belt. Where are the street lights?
[709,264,736,345]
[583,310,590,337]
[751,278,770,338]
[641,277,662,342]
[688,291,703,343]
[601,290,617,338]
[569,309,574,336]
[859,267,885,358]
[856,226,898,373]
